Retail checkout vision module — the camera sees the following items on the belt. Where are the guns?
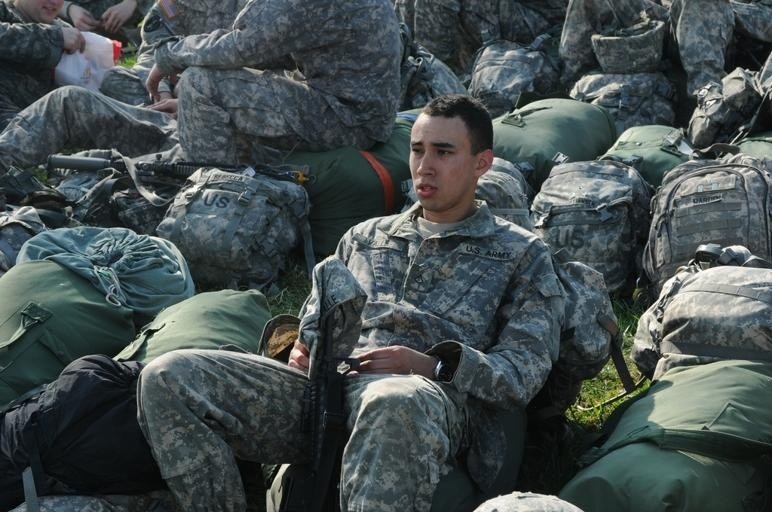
[46,154,317,187]
[278,253,346,510]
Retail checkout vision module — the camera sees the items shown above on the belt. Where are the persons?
[410,0,771,102]
[137,94,571,511]
[2,0,406,199]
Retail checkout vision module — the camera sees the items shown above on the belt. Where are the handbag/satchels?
[0,166,310,512]
[280,40,772,512]
